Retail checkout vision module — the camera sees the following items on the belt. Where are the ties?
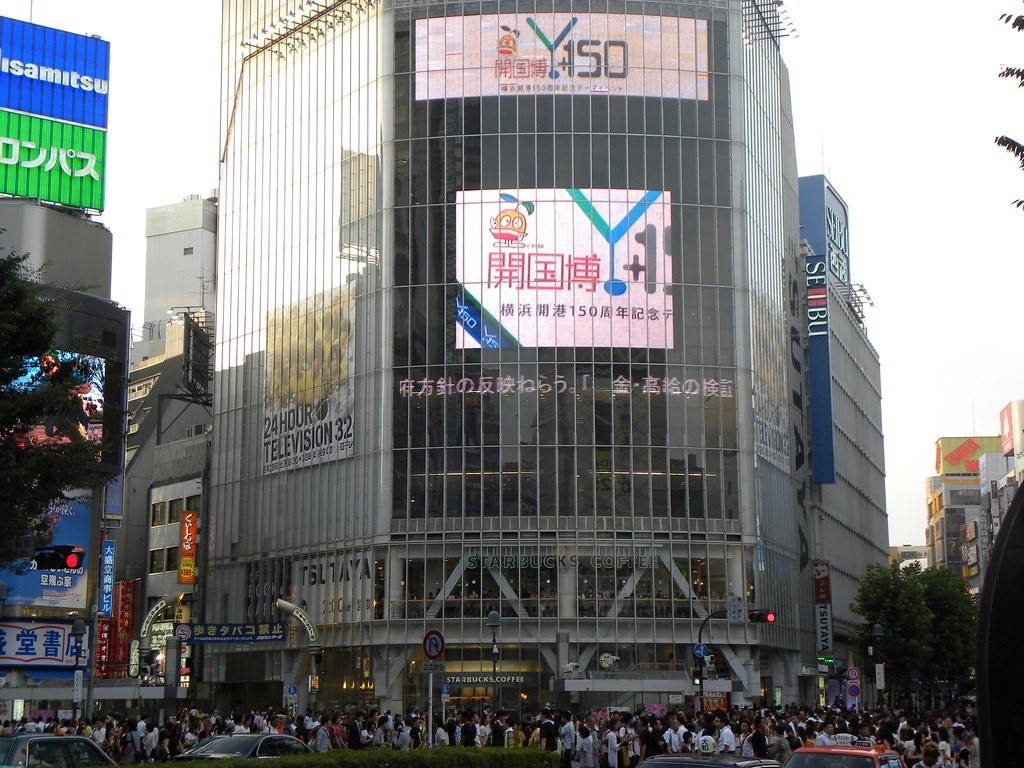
[359,724,361,738]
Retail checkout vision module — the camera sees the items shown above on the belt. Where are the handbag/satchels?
[133,751,143,764]
[569,743,575,759]
[150,748,158,759]
[103,747,110,757]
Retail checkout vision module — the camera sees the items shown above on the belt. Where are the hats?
[354,710,365,719]
[522,713,535,723]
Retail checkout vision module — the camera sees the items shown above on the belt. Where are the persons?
[408,591,757,618]
[0,687,977,768]
[38,355,101,462]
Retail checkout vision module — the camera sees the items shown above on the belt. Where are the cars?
[0,728,118,768]
[781,741,909,768]
[633,752,783,768]
[172,733,318,762]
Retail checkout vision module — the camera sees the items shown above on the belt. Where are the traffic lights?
[748,612,777,623]
[150,663,160,676]
[33,551,86,570]
[692,669,701,686]
[140,665,152,676]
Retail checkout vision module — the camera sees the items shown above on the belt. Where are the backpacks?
[42,724,51,733]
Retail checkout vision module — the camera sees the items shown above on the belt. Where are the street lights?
[485,611,502,716]
[70,618,87,722]
[871,623,886,712]
